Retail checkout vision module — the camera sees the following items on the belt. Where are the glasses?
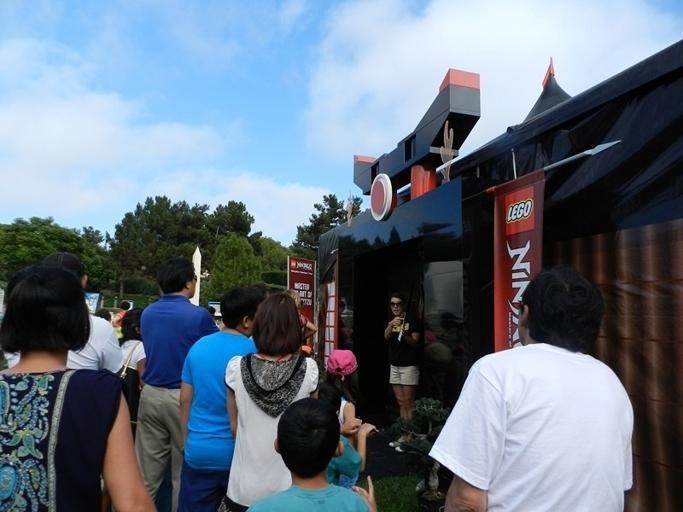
[193,275,197,282]
[389,302,402,306]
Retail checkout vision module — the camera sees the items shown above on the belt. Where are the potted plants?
[398,398,451,512]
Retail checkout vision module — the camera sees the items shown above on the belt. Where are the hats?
[327,350,358,381]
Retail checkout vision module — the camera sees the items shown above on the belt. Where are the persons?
[383,296,420,454]
[217,294,320,512]
[247,397,379,511]
[323,349,377,488]
[1,253,148,443]
[428,268,635,512]
[177,287,266,511]
[134,253,220,511]
[1,266,157,511]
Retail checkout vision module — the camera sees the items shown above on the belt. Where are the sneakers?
[394,445,406,453]
[389,438,406,447]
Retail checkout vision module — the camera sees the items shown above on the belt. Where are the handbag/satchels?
[114,341,143,424]
